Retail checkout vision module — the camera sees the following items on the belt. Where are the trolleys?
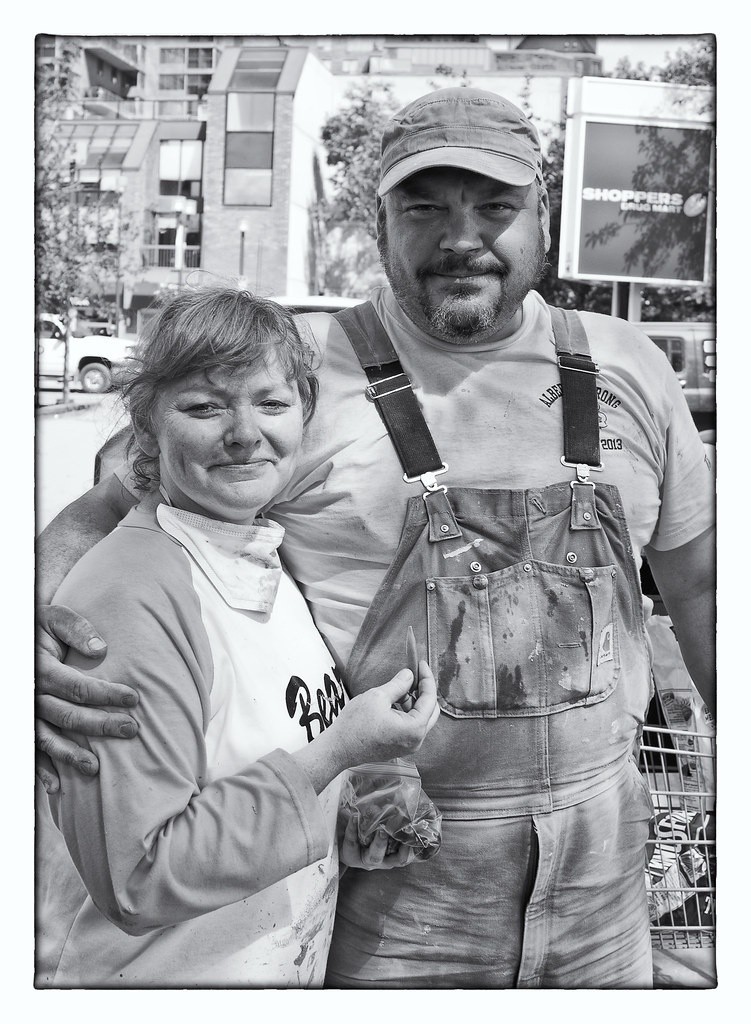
[635,726,716,958]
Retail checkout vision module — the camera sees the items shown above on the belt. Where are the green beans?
[359,798,439,848]
[406,626,418,694]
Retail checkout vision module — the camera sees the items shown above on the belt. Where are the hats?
[375,86,543,191]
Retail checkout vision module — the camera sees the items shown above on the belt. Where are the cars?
[38,314,146,396]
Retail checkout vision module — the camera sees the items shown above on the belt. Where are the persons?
[35,86,715,991]
[35,292,442,991]
